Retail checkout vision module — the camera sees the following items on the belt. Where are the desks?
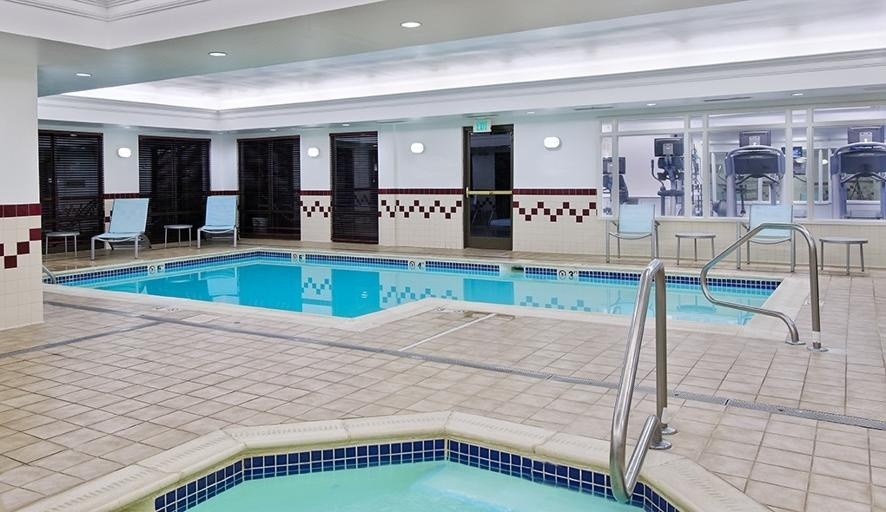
[44,232,79,260]
[676,293,716,314]
[163,224,192,248]
[818,238,867,275]
[675,233,715,265]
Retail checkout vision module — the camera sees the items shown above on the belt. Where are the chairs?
[196,195,239,249]
[605,204,659,264]
[605,286,654,317]
[90,198,151,260]
[736,204,795,272]
[197,267,237,296]
